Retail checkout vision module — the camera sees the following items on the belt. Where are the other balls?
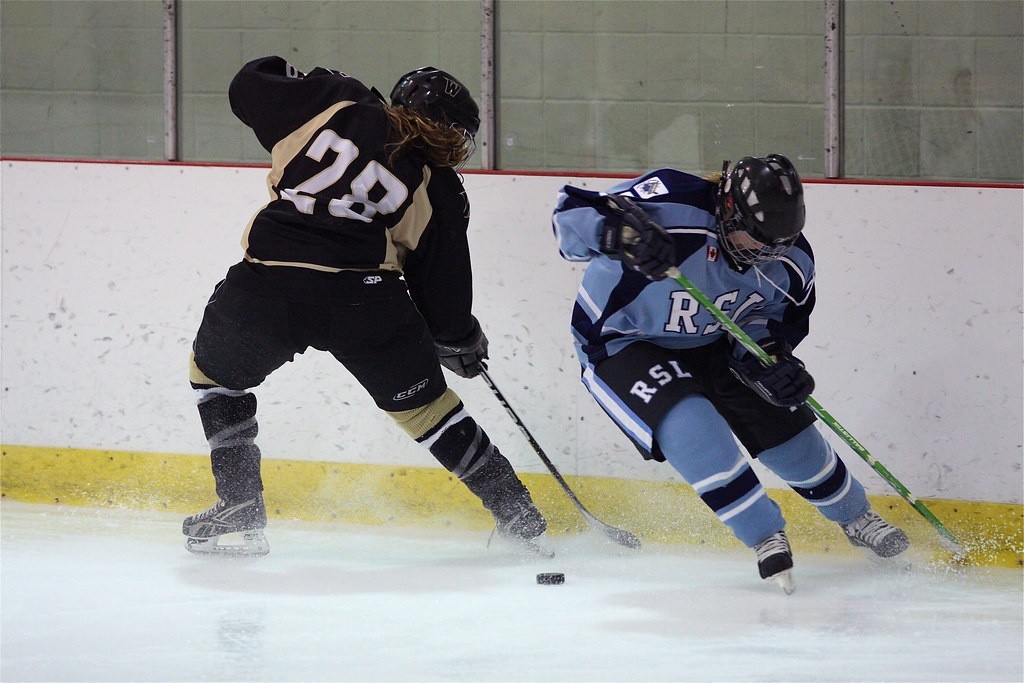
[537,573,565,585]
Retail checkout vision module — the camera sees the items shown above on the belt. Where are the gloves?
[740,337,815,407]
[598,196,681,281]
[433,317,488,379]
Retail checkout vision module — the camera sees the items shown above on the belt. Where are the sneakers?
[181,489,269,556]
[753,528,797,594]
[469,488,555,558]
[841,506,910,568]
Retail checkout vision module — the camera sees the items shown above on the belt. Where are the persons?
[551,154,912,596]
[181,55,554,557]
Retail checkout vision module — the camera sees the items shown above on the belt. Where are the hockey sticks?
[474,362,640,552]
[565,185,958,546]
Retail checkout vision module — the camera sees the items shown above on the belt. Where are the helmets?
[715,153,813,264]
[390,66,479,174]
